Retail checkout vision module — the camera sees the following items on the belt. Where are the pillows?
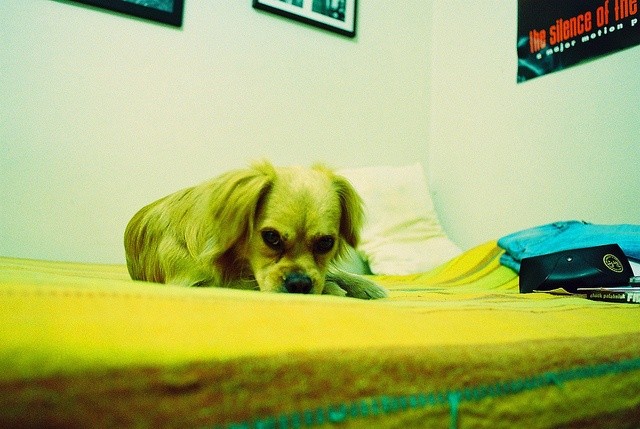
[337,161,462,275]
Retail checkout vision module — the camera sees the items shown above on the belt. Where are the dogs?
[123,158,387,300]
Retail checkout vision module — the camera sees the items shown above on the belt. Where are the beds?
[0,222,640,428]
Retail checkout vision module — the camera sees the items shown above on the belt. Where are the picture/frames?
[251,0,358,38]
[54,0,185,30]
